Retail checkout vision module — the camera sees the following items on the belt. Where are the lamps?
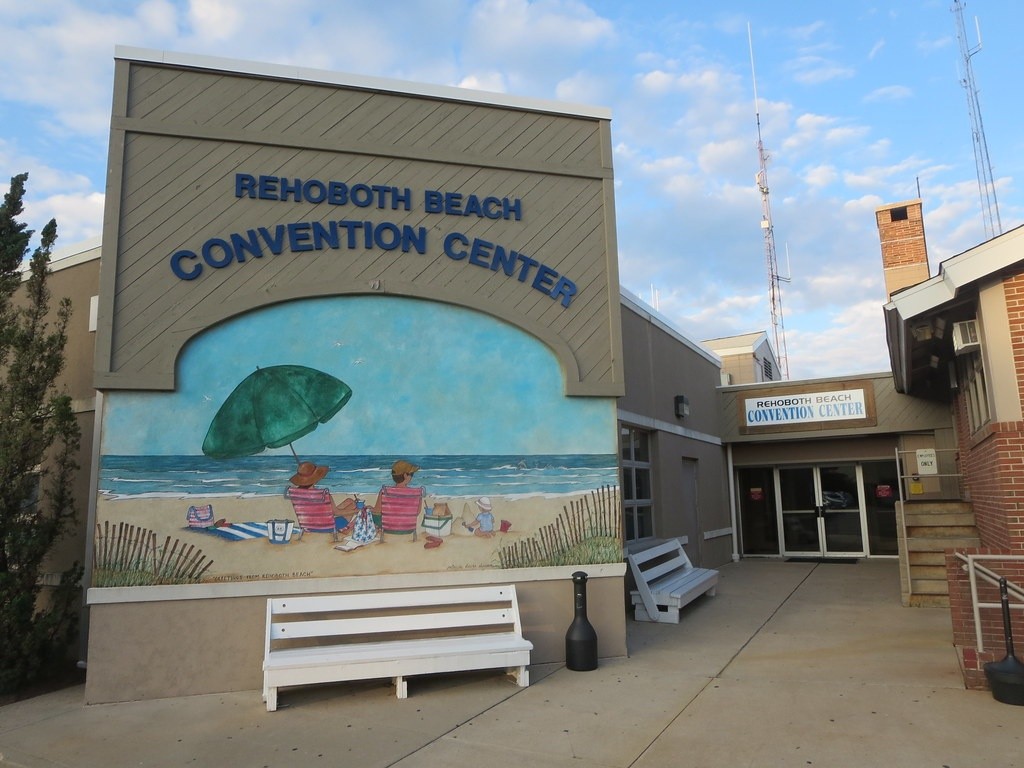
[910,321,935,341]
[674,395,690,418]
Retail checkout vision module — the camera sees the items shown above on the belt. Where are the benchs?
[262,585,534,711]
[626,537,720,623]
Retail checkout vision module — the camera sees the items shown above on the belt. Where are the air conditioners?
[952,320,981,356]
